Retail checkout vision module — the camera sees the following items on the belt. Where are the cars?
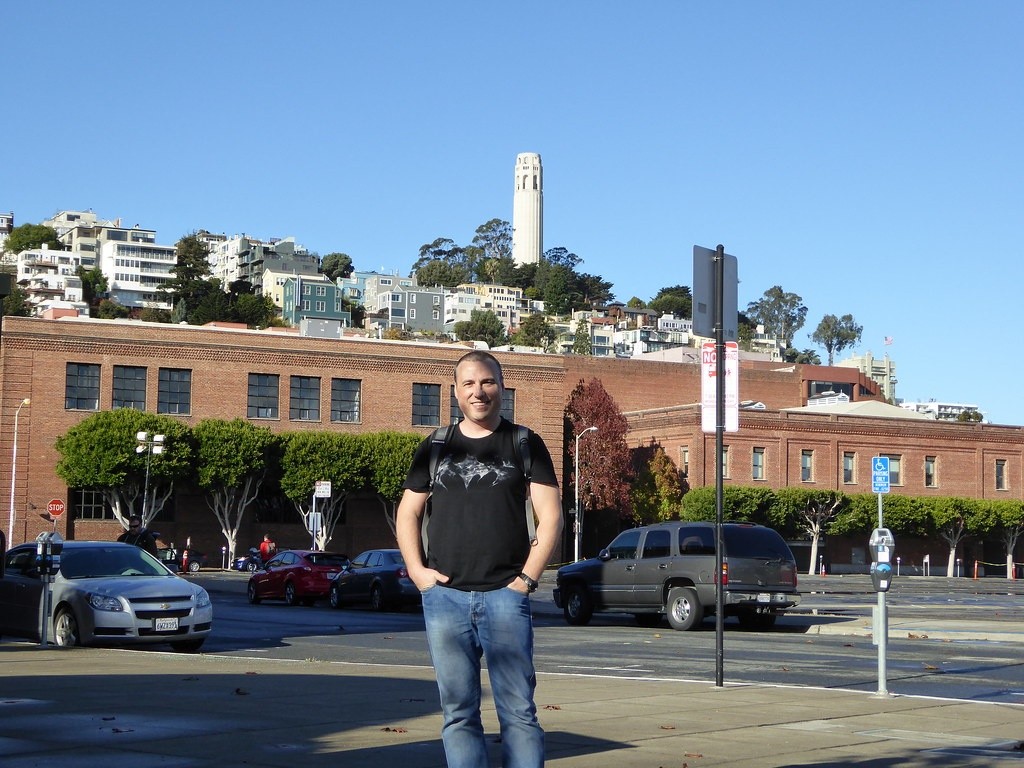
[155,548,208,572]
[330,549,421,613]
[4,540,213,653]
[248,550,350,607]
[231,549,285,573]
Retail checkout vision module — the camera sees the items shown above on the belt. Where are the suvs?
[553,520,802,633]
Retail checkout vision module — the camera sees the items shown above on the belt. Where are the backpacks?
[267,543,276,554]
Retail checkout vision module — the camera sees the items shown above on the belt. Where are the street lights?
[136,431,167,529]
[7,398,30,549]
[573,426,598,563]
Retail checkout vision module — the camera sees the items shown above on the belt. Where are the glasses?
[129,523,140,528]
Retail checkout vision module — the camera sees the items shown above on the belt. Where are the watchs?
[519,573,539,593]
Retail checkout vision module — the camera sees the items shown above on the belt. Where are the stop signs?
[46,498,65,517]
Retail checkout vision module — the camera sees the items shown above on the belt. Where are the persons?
[117,515,157,558]
[396,351,564,768]
[260,534,275,568]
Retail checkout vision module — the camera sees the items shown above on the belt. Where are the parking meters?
[868,528,897,699]
[956,559,960,577]
[36,531,64,649]
[222,546,226,569]
[819,555,823,576]
[897,557,901,576]
